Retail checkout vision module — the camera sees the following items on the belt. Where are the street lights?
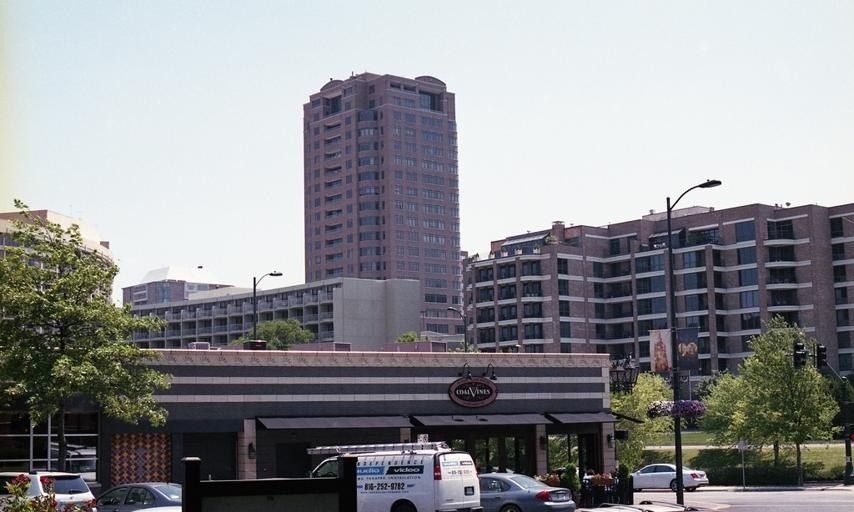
[446,306,468,352]
[665,176,721,504]
[253,271,283,340]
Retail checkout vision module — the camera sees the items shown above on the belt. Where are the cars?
[631,463,710,493]
[82,481,182,512]
[478,471,576,512]
[0,469,98,511]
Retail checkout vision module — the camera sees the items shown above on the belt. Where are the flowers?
[646,400,706,419]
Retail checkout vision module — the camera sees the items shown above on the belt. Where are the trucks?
[305,443,481,512]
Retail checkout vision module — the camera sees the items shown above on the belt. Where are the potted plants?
[561,463,582,509]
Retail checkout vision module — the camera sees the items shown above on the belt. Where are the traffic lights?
[816,343,827,369]
[794,341,806,370]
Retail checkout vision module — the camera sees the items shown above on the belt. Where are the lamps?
[608,433,615,448]
[540,435,548,450]
[609,353,639,395]
[459,363,472,380]
[483,362,497,380]
[249,441,255,459]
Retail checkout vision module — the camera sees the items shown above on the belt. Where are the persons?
[582,467,620,507]
[653,331,668,375]
